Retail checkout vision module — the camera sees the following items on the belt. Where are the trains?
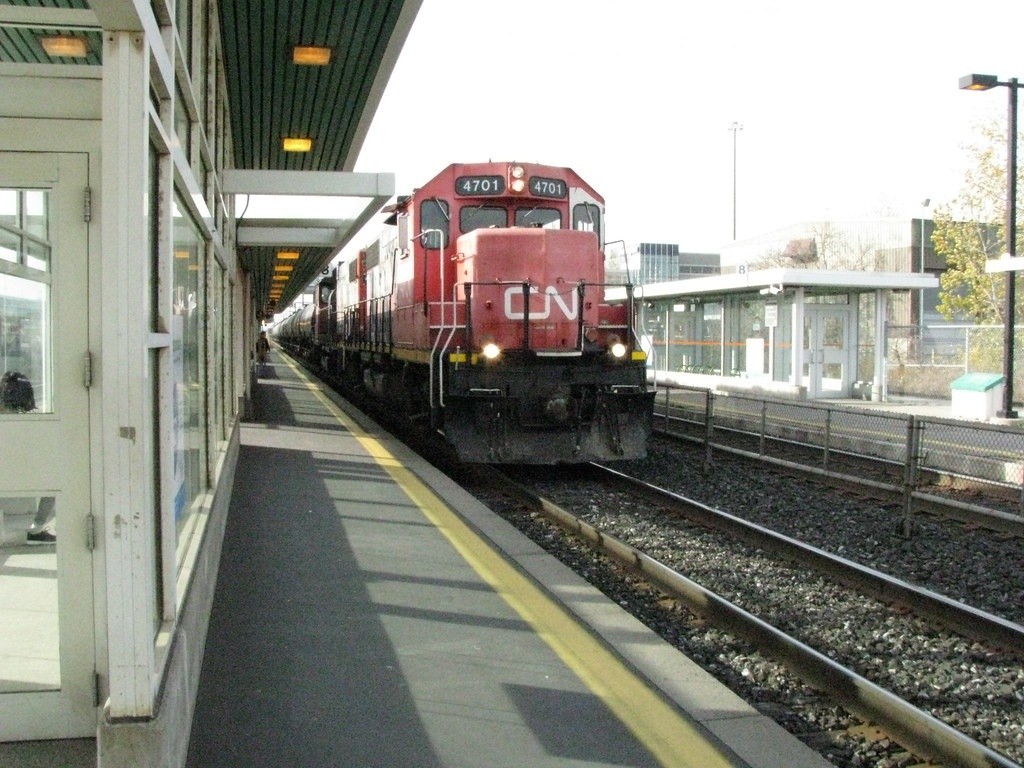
[267,159,659,464]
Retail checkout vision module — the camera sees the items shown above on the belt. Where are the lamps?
[292,46,331,66]
[40,35,94,59]
[284,138,312,151]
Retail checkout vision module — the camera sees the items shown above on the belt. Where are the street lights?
[728,121,745,241]
[958,72,1024,420]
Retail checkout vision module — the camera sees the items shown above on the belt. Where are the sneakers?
[26,527,56,545]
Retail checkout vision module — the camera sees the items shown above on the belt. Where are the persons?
[0,371,56,544]
[257,331,270,372]
